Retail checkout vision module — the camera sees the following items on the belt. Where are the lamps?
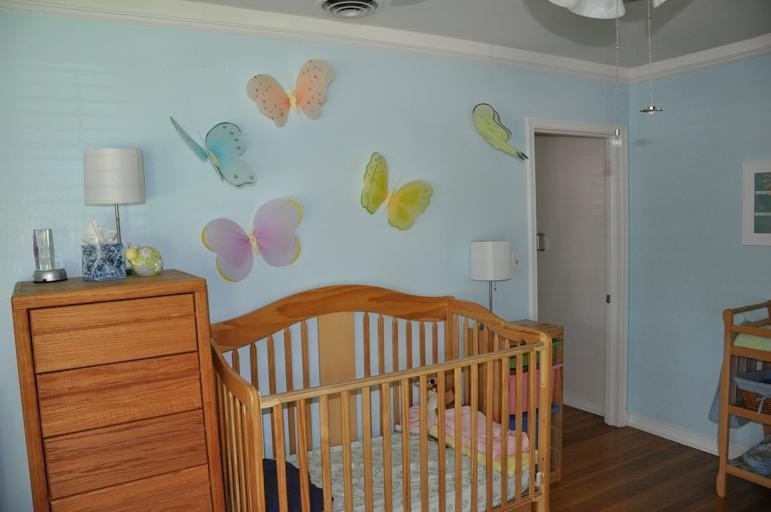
[82,144,147,278]
[469,238,514,350]
[607,0,667,152]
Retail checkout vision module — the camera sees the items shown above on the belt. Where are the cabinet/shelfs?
[467,319,567,487]
[7,268,226,511]
[714,303,769,505]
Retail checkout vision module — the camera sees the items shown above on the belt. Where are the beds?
[209,284,557,511]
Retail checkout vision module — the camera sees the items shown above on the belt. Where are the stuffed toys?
[415,368,454,415]
